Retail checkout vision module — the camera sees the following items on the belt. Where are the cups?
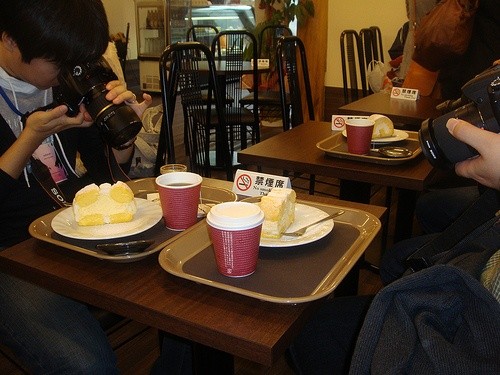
[155,172,203,231]
[206,202,265,278]
[345,119,375,156]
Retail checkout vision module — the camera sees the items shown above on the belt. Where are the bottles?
[146,9,161,28]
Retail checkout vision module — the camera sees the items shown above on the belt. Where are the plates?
[342,129,409,145]
[254,203,334,247]
[50,197,163,240]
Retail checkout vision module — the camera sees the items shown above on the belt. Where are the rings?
[126,93,136,103]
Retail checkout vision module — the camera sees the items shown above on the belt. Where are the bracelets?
[114,137,137,151]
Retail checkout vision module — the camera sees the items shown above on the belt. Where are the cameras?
[56,55,143,146]
[419,64,500,170]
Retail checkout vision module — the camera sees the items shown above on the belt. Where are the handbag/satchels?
[412,0,479,72]
[387,21,409,60]
[367,60,392,95]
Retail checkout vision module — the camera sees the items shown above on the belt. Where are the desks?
[236,120,433,305]
[339,86,431,126]
[0,175,388,375]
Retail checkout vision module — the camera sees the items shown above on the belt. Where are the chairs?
[132,21,316,195]
[339,27,383,102]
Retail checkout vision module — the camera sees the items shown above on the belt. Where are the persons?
[0,0,153,375]
[284,60,500,375]
[388,0,409,67]
[403,0,500,99]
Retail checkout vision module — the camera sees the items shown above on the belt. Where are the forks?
[281,210,345,236]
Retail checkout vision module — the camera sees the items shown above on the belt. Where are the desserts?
[369,114,394,138]
[258,188,296,238]
[73,181,136,225]
